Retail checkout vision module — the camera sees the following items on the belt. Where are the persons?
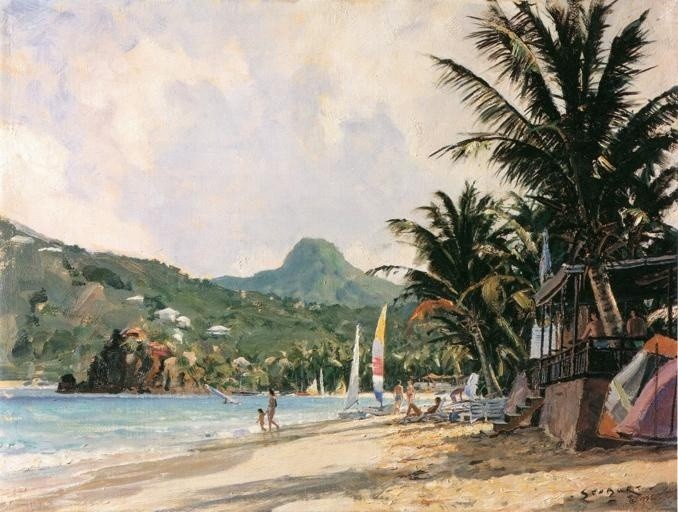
[266,388,280,432]
[449,387,465,403]
[392,380,403,416]
[405,378,415,405]
[581,311,609,349]
[405,396,441,416]
[256,407,267,431]
[627,307,648,350]
